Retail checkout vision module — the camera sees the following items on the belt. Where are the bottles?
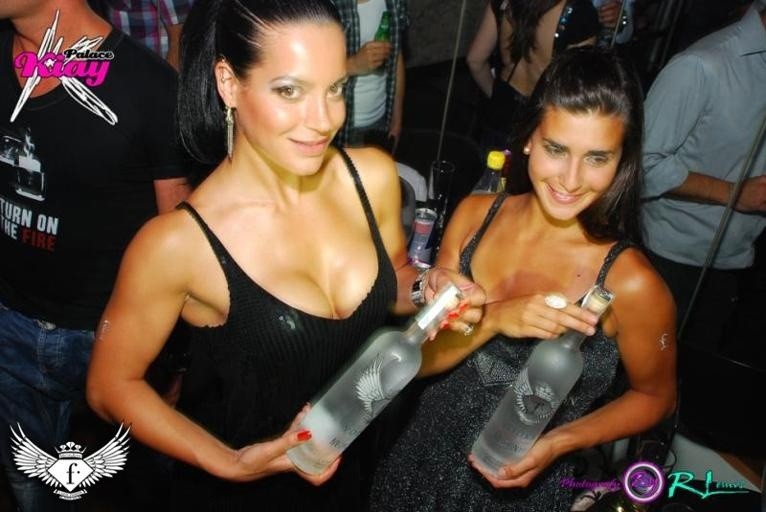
[466,147,510,196]
[283,281,464,477]
[369,8,392,78]
[470,283,616,483]
[431,193,452,264]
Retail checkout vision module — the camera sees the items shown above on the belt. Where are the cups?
[426,156,454,200]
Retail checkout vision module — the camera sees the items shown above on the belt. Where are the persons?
[0,0,764,511]
